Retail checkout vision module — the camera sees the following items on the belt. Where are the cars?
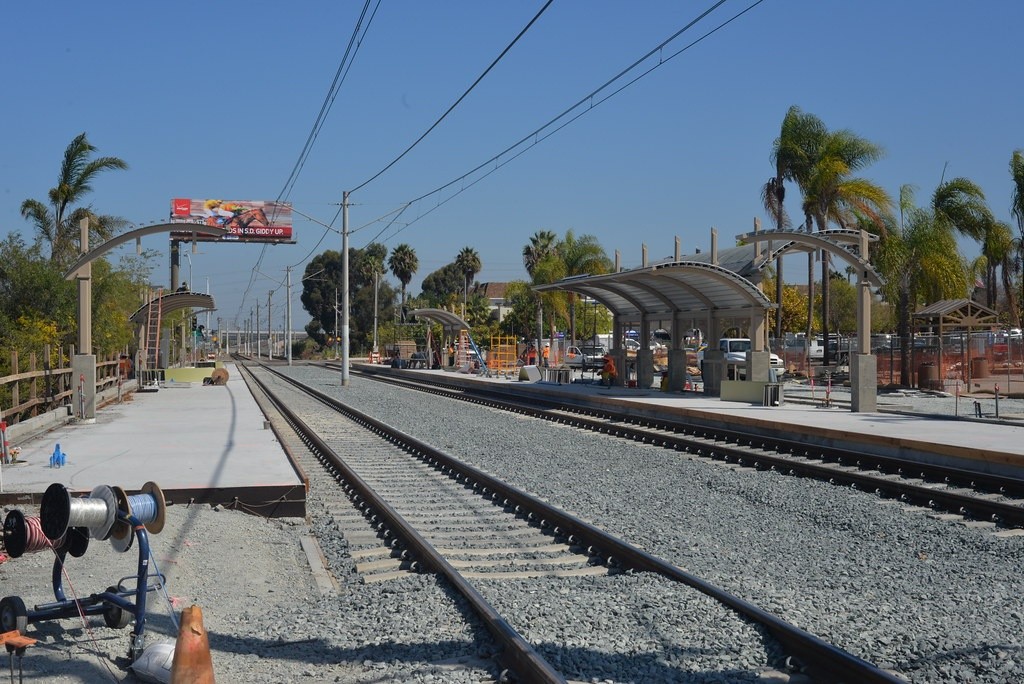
[697,336,786,382]
[1009,328,1022,340]
[622,338,641,350]
[788,332,828,360]
[565,345,609,372]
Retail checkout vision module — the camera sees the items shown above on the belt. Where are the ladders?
[144,287,161,369]
[455,329,471,368]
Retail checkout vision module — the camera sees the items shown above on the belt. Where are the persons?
[449,343,455,366]
[599,356,618,388]
[541,343,550,368]
[207,200,257,228]
[529,345,536,365]
[570,349,575,358]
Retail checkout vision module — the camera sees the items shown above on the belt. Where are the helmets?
[545,343,550,346]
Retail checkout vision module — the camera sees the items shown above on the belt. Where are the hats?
[602,355,609,360]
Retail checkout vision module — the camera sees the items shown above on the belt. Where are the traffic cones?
[168,604,217,684]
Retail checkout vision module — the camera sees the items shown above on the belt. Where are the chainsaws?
[203,368,229,386]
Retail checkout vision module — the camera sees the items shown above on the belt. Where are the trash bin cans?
[918,362,938,388]
[972,357,989,377]
